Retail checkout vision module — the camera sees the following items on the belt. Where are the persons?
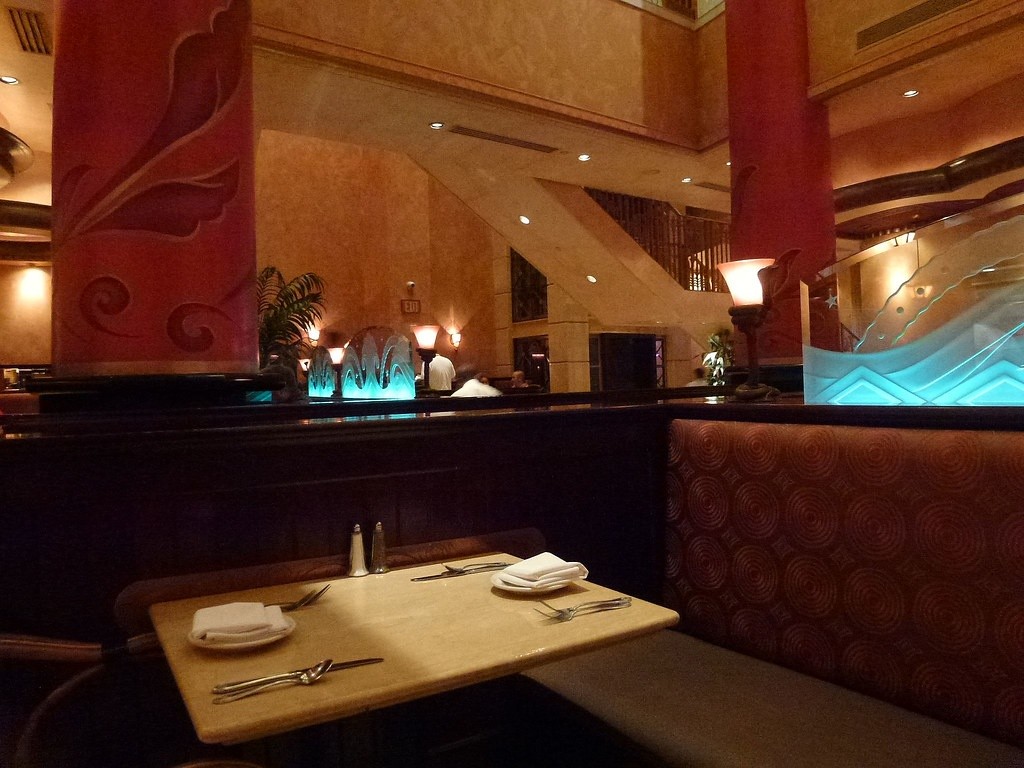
[510,371,531,388]
[415,346,456,390]
[260,354,299,403]
[683,368,710,387]
[473,373,490,386]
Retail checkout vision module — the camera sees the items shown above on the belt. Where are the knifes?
[411,564,514,581]
[212,658,384,694]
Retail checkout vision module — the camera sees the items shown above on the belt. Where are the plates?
[491,572,571,594]
[187,614,296,649]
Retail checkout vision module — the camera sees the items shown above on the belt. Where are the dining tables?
[147,554,680,768]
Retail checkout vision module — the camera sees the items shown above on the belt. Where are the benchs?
[511,420,1024,768]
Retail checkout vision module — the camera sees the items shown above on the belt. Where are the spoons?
[213,659,333,703]
[442,561,506,572]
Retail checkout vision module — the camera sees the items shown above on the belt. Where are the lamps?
[715,249,802,404]
[518,215,531,225]
[586,275,597,283]
[412,326,441,398]
[427,122,445,130]
[299,316,348,387]
[902,90,920,98]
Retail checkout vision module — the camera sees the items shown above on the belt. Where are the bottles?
[348,524,369,576]
[370,522,389,573]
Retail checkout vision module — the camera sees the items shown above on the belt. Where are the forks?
[538,596,631,614]
[264,583,332,607]
[533,602,631,622]
[279,590,317,611]
